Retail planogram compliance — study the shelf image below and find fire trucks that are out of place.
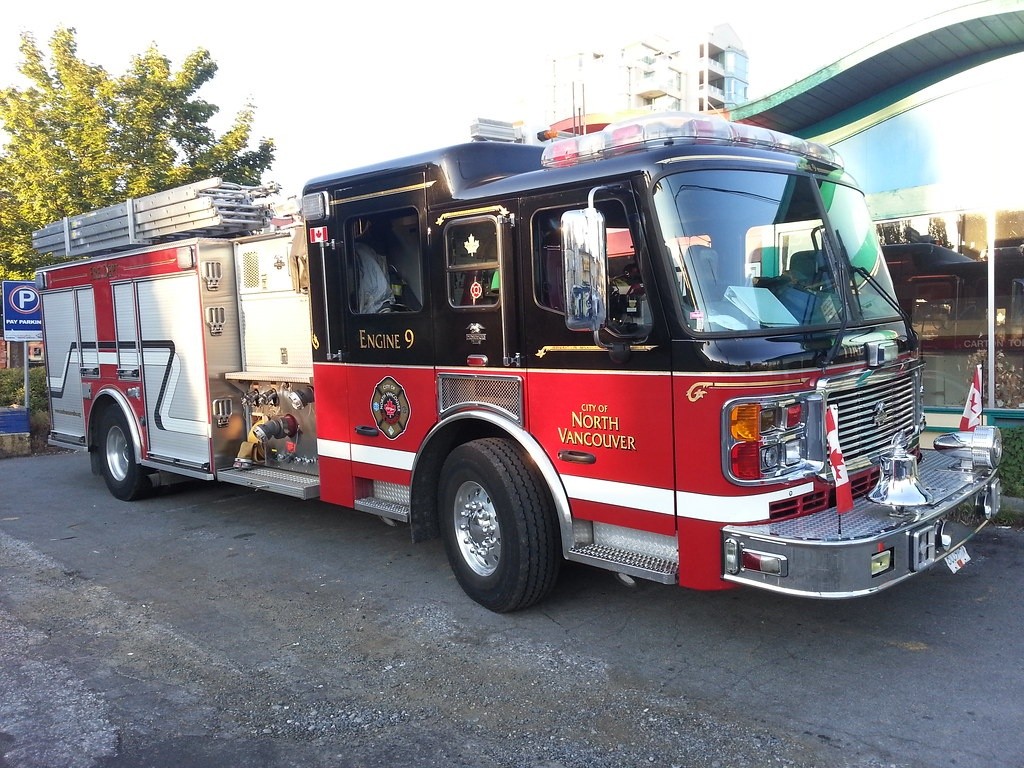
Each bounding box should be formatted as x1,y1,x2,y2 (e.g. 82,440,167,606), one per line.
29,110,1005,620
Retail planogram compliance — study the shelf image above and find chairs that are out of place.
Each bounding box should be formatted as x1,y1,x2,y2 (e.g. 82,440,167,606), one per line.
685,244,725,303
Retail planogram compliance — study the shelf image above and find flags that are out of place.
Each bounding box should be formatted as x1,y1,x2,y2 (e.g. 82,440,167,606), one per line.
824,404,854,516
960,363,983,432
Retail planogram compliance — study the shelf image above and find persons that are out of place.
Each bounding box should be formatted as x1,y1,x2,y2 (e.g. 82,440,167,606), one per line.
609,263,643,313
